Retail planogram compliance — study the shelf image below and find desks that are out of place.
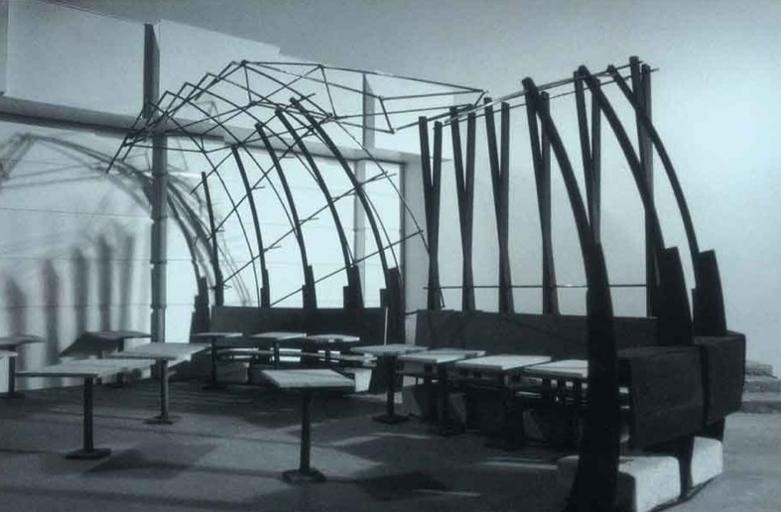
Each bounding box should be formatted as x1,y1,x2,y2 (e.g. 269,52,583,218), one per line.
195,333,363,398
2,331,46,400
398,331,588,460
259,369,355,484
89,331,153,390
16,356,157,459
108,341,212,425
349,343,429,425
